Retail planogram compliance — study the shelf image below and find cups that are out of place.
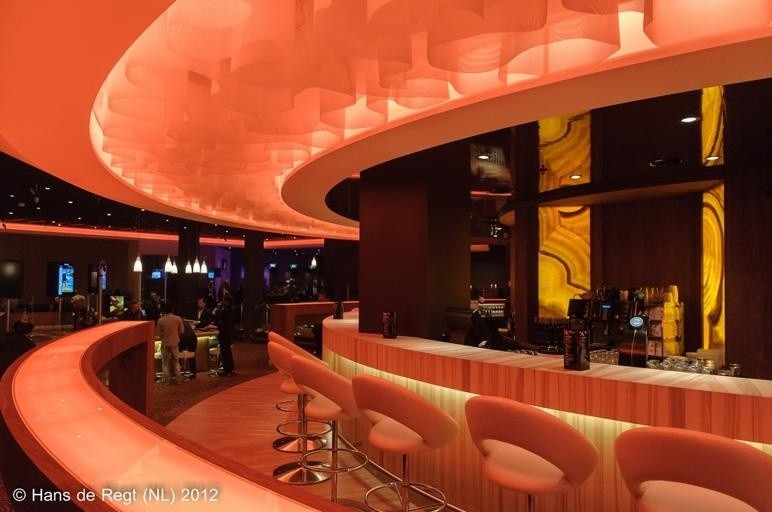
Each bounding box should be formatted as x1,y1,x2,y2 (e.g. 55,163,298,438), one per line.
589,348,619,365
646,356,716,375
383,311,397,338
619,283,679,304
562,328,590,370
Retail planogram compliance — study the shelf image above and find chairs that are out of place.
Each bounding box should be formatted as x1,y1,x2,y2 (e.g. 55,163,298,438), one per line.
349,371,462,511
464,393,602,511
292,356,368,511
267,328,328,455
616,419,772,511
265,341,332,485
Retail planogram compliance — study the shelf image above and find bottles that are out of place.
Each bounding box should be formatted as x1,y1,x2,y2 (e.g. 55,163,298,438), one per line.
728,363,742,376
333,298,343,320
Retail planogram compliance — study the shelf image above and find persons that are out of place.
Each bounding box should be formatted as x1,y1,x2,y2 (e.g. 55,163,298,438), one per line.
119,292,235,385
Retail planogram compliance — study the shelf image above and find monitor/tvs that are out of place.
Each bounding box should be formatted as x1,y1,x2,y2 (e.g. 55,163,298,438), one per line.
207,271,215,280
445,307,487,337
151,269,164,280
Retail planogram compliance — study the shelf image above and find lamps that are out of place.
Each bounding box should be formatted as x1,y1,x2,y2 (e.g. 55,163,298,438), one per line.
133,236,208,274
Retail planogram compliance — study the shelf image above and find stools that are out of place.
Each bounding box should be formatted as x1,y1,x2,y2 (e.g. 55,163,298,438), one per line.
208,347,225,377
173,351,196,378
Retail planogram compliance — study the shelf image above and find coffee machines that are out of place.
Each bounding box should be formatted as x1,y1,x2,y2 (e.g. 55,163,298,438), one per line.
567,298,686,367
